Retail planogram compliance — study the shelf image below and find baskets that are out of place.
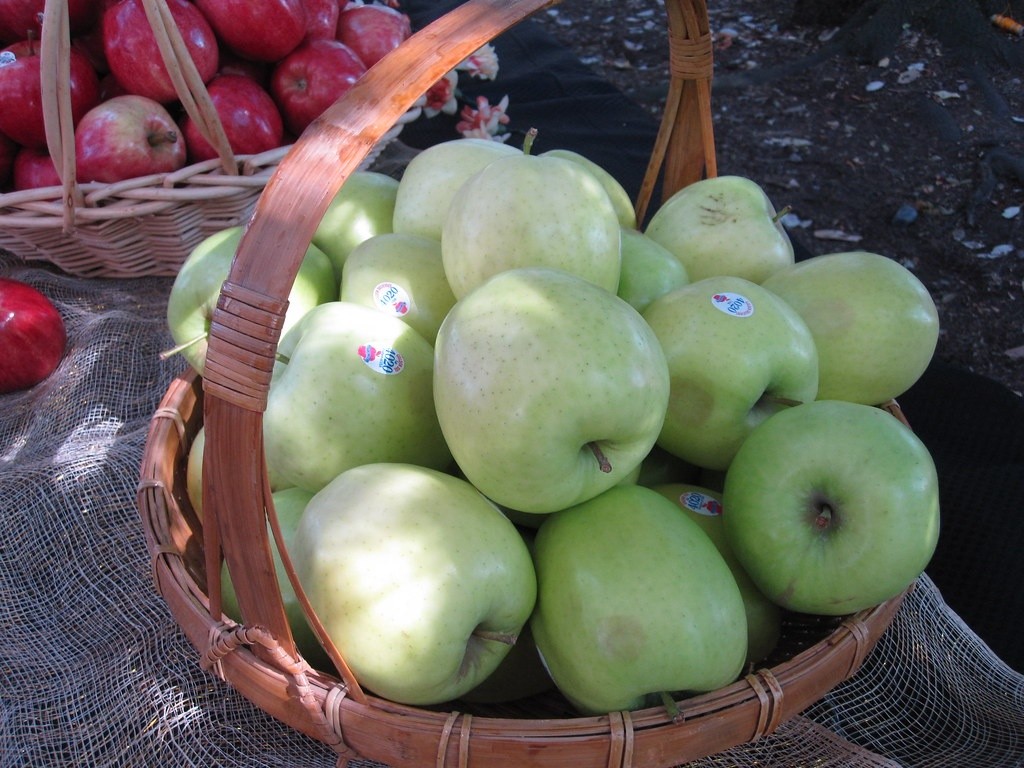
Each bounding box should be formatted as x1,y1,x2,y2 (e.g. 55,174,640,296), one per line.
0,0,427,280
137,0,911,768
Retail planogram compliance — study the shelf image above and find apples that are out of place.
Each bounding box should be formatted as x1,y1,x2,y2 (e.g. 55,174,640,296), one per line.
0,0,413,196
0,276,65,394
167,127,942,724
